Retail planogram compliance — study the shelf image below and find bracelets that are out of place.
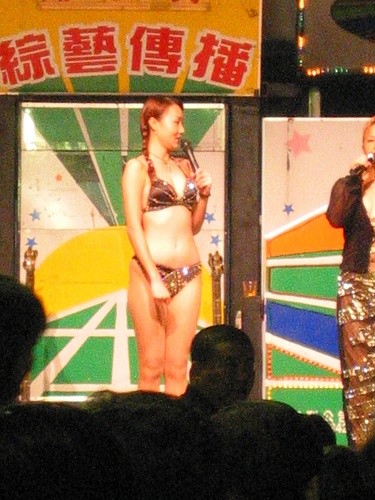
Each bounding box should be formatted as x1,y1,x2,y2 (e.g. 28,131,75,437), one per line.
199,193,211,198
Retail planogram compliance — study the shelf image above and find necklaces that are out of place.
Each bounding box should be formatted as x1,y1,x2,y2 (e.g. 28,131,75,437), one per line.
149,151,172,168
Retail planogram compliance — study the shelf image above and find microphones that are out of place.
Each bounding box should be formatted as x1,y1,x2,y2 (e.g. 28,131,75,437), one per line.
180,138,199,173
356,153,375,176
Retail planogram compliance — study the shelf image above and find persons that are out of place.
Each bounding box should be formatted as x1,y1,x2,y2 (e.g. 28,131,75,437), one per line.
325,116,375,449
121,96,211,397
0,273,375,500
